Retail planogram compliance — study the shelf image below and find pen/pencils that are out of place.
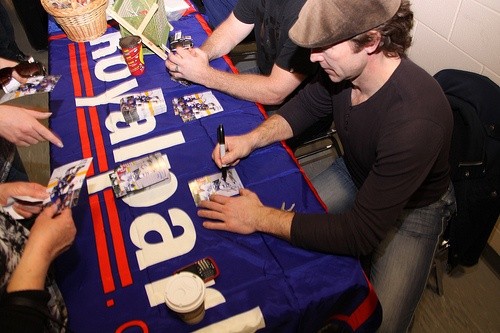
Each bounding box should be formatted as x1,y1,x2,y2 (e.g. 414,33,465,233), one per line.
217,123,227,183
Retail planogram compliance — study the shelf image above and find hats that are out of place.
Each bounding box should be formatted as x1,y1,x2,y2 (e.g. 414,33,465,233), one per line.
288,0,402,48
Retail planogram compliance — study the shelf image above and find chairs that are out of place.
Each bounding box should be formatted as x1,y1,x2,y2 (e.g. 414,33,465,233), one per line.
295,68,500,298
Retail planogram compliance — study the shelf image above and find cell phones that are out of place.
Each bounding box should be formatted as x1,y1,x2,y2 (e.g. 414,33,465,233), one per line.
173,257,219,283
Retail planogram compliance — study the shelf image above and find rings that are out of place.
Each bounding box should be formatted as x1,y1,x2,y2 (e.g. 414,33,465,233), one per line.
175,65,179,73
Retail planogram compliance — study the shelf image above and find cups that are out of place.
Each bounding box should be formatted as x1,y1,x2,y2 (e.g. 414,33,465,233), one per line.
119,36,146,77
164,271,206,325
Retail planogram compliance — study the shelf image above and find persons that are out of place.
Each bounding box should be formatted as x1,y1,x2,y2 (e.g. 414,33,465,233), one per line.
0,57,63,231
0,181,77,333
165,0,336,156
195,0,457,333
0,0,48,63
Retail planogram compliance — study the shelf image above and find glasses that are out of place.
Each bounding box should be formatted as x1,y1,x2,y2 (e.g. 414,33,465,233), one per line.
0,59,47,90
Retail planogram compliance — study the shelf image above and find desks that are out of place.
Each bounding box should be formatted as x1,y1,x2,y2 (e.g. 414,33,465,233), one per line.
44,0,385,333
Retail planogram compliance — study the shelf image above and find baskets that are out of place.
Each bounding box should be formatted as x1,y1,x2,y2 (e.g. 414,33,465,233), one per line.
40,0,110,43
